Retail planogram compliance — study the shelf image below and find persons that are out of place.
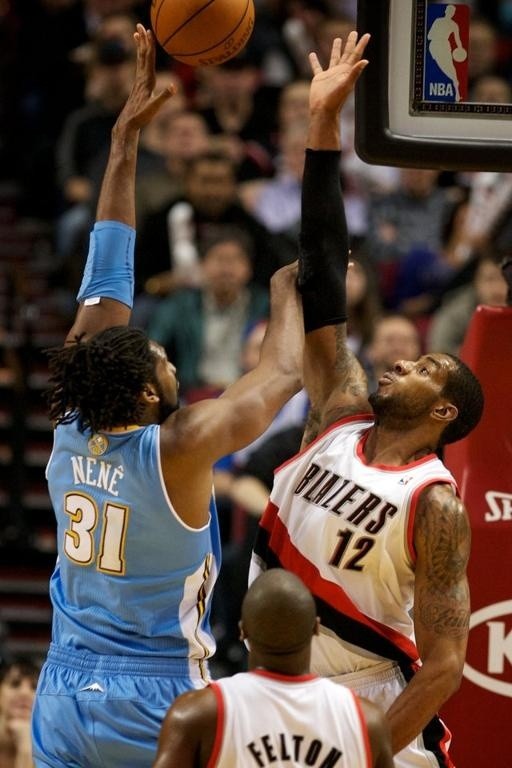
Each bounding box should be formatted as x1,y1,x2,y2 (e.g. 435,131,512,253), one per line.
1,2,512,768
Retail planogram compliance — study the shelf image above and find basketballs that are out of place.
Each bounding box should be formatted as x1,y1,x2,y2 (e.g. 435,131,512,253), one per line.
149,0,256,67
